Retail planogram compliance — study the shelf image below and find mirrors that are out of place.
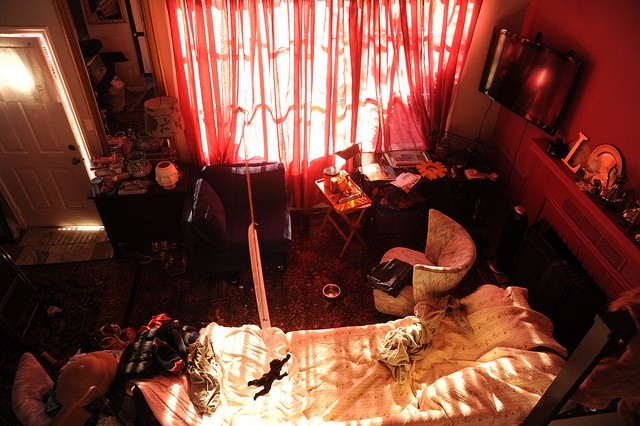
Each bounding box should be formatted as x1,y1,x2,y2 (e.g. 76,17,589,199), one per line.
53,0,189,164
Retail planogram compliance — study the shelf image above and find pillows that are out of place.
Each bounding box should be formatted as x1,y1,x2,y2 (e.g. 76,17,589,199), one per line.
188,179,227,242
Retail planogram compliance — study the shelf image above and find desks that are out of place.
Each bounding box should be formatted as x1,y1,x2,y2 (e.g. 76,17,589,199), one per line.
355,151,500,238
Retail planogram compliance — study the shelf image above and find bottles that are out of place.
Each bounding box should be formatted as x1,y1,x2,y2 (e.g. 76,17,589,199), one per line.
109,167,121,189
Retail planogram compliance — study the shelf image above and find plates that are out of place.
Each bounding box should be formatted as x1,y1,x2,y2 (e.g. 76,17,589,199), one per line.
587,143,625,184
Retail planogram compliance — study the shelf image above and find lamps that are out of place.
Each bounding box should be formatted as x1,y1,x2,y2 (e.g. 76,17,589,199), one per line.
142,97,185,159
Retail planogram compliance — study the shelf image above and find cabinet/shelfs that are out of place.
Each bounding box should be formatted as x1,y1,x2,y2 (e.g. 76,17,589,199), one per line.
89,163,192,260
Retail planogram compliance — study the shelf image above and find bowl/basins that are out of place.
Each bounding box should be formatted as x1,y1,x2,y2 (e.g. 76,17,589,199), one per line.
323,284,342,298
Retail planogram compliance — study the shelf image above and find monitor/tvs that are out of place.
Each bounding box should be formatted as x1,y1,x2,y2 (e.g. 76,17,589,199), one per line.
480,28,585,137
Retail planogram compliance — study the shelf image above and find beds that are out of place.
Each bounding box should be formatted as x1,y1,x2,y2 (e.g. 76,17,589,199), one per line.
127,284,564,426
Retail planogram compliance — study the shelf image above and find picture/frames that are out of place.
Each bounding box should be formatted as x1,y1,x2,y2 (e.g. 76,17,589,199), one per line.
82,0,127,25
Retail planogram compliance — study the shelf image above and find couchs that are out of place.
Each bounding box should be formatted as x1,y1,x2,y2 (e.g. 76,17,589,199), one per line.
373,208,476,316
182,162,292,274
